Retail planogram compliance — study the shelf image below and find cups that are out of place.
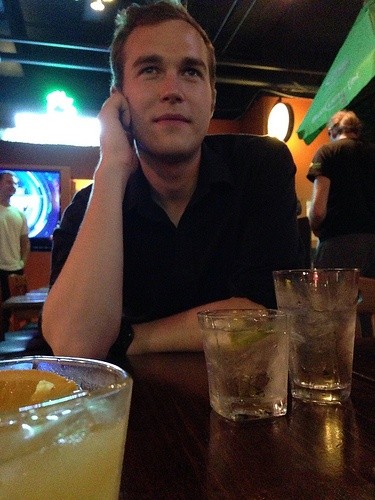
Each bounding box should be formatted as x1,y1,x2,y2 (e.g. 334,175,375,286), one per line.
272,267,362,406
196,307,291,422
0,355,134,500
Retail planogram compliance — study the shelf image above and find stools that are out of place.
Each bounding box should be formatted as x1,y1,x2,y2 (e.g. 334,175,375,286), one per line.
0,331,34,354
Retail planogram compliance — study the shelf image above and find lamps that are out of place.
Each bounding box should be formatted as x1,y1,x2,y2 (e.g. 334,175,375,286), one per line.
266,100,293,144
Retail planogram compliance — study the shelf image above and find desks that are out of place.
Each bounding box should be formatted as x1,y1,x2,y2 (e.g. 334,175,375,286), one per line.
3,286,49,310
106,354,375,500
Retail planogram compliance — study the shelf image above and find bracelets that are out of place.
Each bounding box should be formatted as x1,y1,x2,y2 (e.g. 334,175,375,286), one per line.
106,315,135,362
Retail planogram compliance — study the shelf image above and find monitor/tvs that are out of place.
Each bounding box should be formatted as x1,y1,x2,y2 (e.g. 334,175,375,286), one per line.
0,164,71,252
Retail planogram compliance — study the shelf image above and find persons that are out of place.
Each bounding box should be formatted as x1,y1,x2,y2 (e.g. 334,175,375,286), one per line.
0,170,30,340
38,0,308,359
305,110,375,280
